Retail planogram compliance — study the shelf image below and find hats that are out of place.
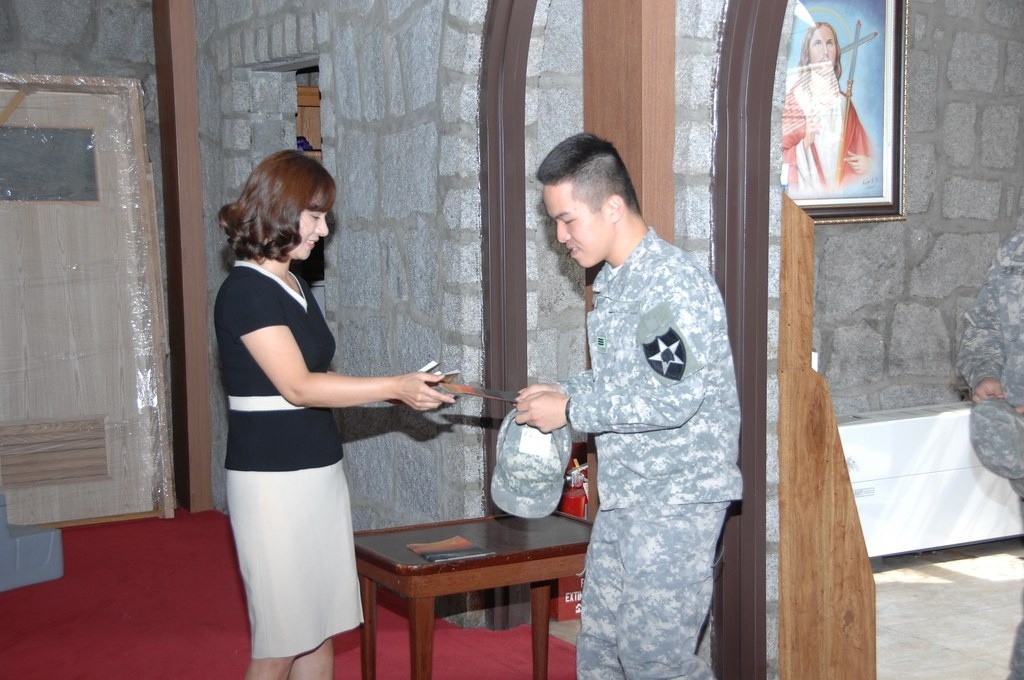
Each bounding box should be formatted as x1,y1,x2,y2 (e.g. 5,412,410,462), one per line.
490,408,572,519
969,399,1024,498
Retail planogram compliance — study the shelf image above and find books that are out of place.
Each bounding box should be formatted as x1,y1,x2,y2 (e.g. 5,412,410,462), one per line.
438,382,519,405
406,534,496,563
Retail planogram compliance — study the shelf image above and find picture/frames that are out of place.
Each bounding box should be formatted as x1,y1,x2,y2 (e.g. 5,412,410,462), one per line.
780,1,910,227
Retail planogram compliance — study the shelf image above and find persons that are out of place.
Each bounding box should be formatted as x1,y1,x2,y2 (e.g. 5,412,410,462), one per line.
213,151,457,679
955,207,1024,678
513,133,747,680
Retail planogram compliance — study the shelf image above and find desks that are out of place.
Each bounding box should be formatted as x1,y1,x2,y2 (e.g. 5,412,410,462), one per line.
353,510,594,680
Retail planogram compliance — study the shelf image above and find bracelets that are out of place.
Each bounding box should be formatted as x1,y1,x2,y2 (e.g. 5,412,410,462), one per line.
565,397,572,425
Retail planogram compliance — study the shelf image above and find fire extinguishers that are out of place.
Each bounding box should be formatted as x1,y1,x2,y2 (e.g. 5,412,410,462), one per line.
558,463,589,521
